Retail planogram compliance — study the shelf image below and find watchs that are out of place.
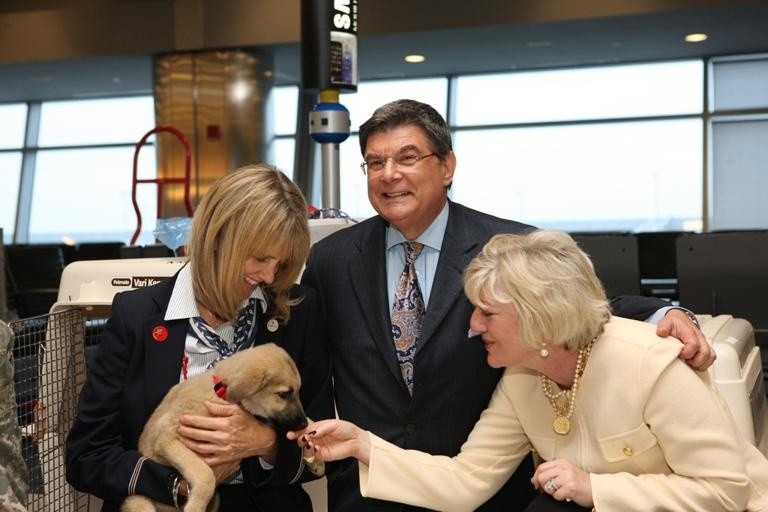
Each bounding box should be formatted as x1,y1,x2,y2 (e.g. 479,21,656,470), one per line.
685,311,698,327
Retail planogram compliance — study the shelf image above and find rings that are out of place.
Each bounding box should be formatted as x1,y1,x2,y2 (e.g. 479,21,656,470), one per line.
549,479,558,492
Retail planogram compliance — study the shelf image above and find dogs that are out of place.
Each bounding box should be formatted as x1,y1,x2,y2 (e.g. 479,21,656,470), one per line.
119,341,308,512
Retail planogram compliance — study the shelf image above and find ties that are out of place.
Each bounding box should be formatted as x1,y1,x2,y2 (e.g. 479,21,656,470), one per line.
391,242,425,396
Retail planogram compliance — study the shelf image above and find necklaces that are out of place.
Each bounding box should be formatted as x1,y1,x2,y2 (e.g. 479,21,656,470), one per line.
541,332,597,435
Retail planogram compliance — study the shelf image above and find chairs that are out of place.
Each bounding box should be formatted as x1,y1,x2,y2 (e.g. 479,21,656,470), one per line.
2,242,175,319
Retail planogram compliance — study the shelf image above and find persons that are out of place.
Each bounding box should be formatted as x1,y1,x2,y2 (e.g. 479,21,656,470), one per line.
302,100,716,512
287,231,768,511
64,165,334,512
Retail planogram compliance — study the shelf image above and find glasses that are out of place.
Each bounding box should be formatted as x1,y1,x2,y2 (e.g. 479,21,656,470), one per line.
360,153,436,176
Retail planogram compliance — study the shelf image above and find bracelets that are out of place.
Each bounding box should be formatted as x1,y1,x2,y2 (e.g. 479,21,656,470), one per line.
172,473,182,509
